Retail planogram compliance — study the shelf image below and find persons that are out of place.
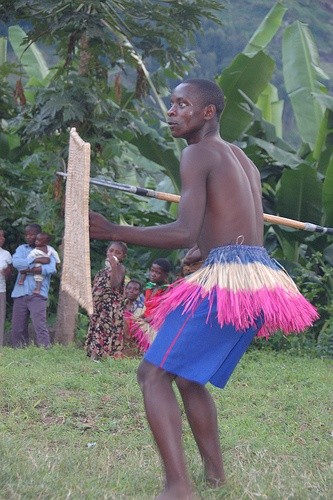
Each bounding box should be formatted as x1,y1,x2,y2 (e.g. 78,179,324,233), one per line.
57,80,333,500
0,224,12,347
18,232,51,295
12,223,61,349
85,241,128,360
125,259,170,356
